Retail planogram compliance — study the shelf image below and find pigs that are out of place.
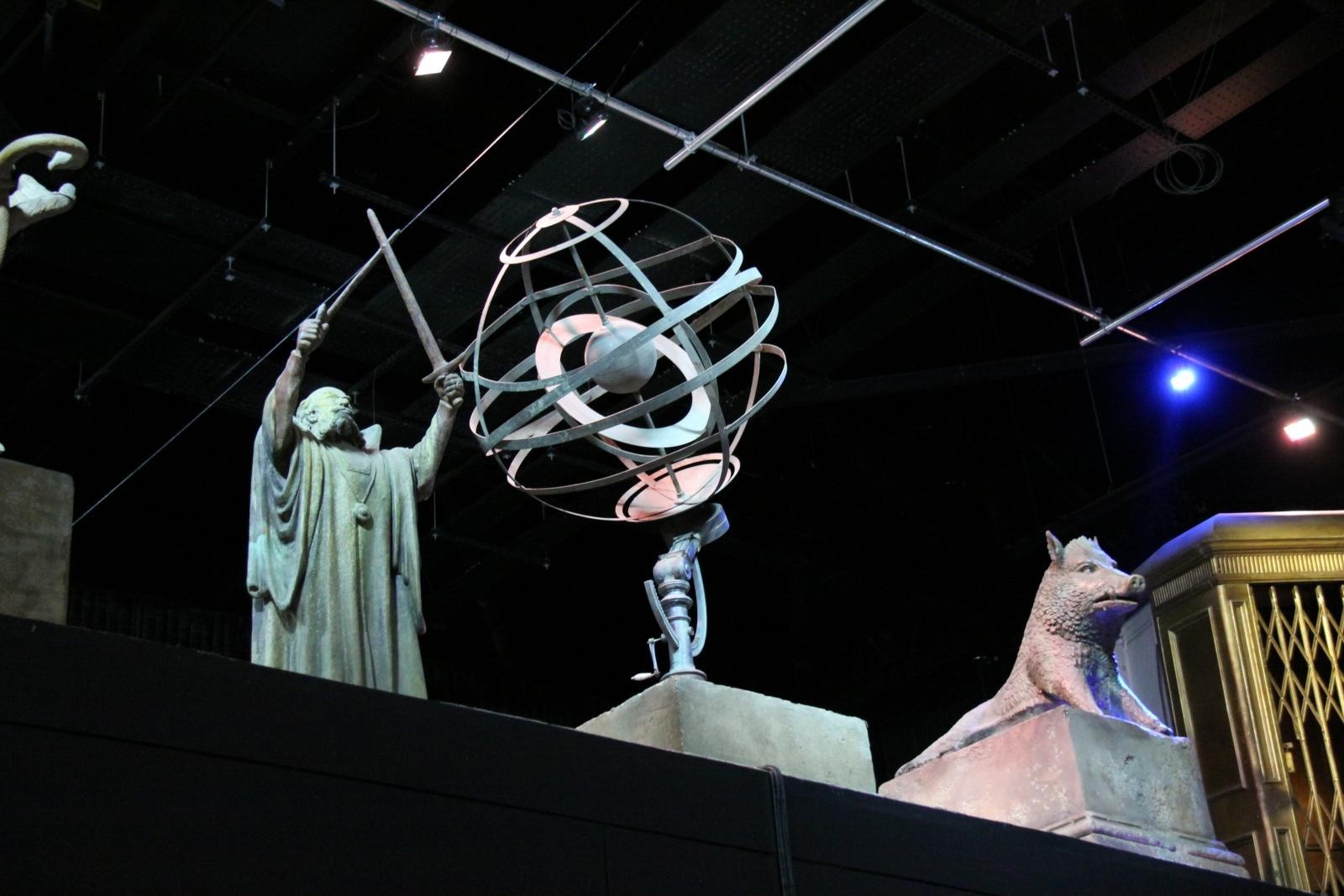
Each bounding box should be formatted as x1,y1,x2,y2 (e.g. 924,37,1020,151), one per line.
894,530,1175,777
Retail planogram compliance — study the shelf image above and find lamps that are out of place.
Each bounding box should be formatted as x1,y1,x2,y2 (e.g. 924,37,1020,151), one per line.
411,13,453,76
557,77,610,141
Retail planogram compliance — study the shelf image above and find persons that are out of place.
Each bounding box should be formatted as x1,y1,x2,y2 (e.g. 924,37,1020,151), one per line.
244,316,466,703
